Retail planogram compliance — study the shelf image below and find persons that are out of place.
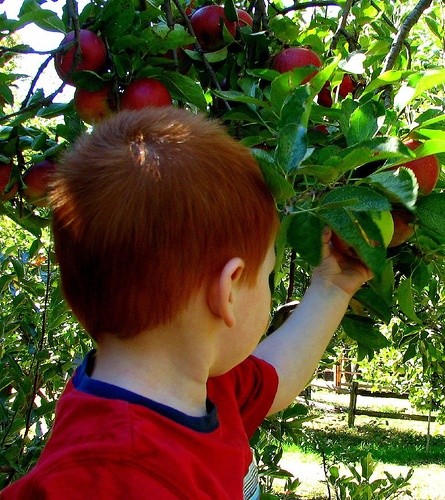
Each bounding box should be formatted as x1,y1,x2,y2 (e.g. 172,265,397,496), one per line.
0,107,375,500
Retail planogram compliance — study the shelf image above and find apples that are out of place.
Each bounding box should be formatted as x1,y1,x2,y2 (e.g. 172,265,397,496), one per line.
74,83,117,125
186,6,253,52
273,47,355,134
0,160,20,200
119,77,172,114
22,160,63,207
328,140,439,258
55,29,104,87
